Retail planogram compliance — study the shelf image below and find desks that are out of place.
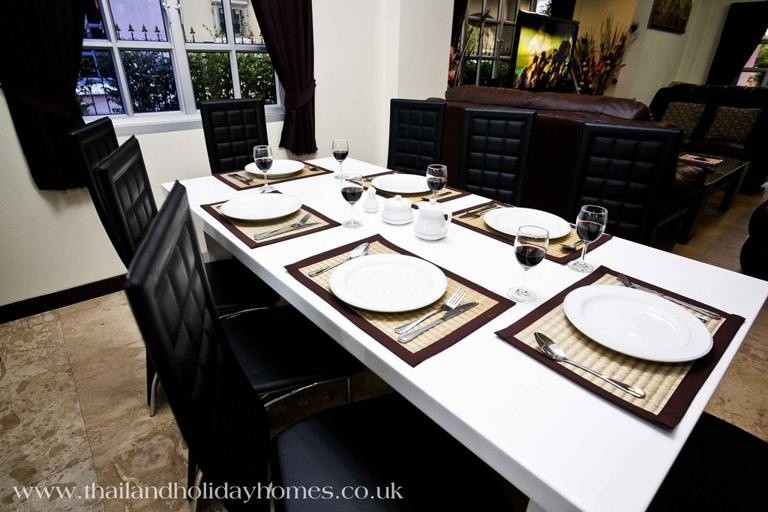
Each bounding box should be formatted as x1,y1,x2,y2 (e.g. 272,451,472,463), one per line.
641,129,751,208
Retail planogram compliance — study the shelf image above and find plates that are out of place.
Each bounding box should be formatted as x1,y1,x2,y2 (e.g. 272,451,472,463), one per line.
220,158,714,362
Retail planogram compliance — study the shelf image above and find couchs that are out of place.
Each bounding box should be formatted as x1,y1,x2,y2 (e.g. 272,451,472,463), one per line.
447,92,707,238
738,200,768,279
646,80,764,193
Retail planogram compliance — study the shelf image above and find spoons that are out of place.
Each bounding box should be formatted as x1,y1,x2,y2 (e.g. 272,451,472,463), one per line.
532,331,646,398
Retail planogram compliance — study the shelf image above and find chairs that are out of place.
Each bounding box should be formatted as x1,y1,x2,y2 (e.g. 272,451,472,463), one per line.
645,399,768,512
111,178,390,512
197,97,266,174
388,100,444,176
92,133,163,267
463,104,535,209
69,113,117,186
583,122,679,257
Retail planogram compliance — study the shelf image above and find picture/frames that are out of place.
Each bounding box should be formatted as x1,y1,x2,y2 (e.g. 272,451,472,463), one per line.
647,1,691,34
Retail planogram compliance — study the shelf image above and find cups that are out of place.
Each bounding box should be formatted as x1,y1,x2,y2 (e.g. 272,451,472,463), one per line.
364,184,452,241
